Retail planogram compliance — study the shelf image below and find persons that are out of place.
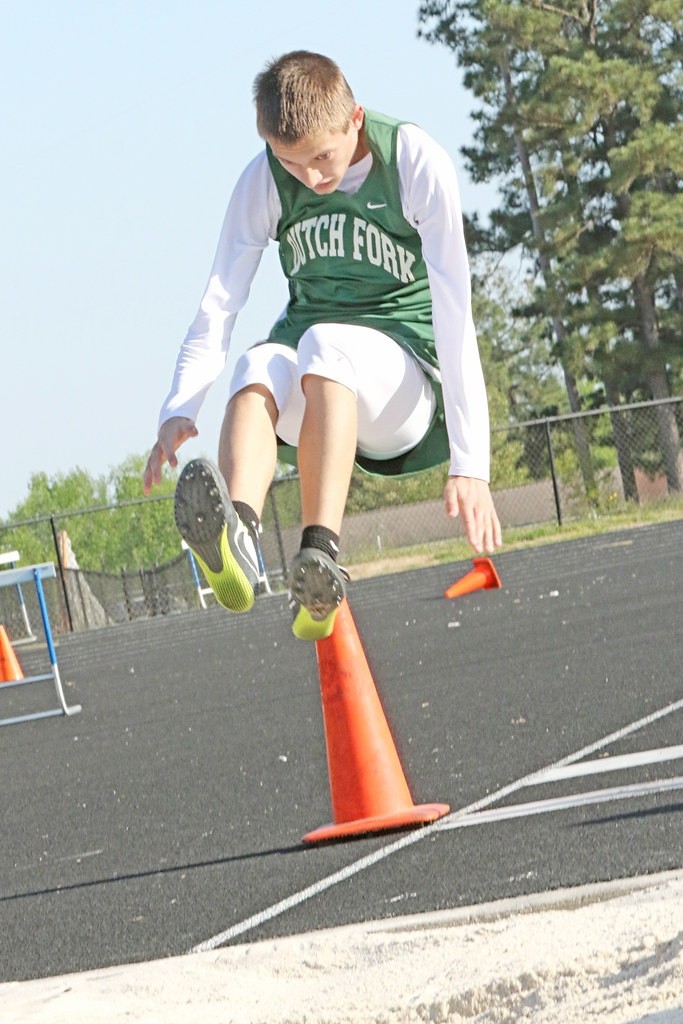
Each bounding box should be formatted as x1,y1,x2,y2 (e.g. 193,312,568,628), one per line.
144,51,502,639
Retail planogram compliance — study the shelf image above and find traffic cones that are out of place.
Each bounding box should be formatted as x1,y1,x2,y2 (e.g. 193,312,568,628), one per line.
444,557,504,599
302,597,450,844
0,625,26,684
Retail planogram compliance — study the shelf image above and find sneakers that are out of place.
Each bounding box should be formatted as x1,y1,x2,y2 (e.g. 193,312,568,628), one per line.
284,550,345,637
178,458,273,614
